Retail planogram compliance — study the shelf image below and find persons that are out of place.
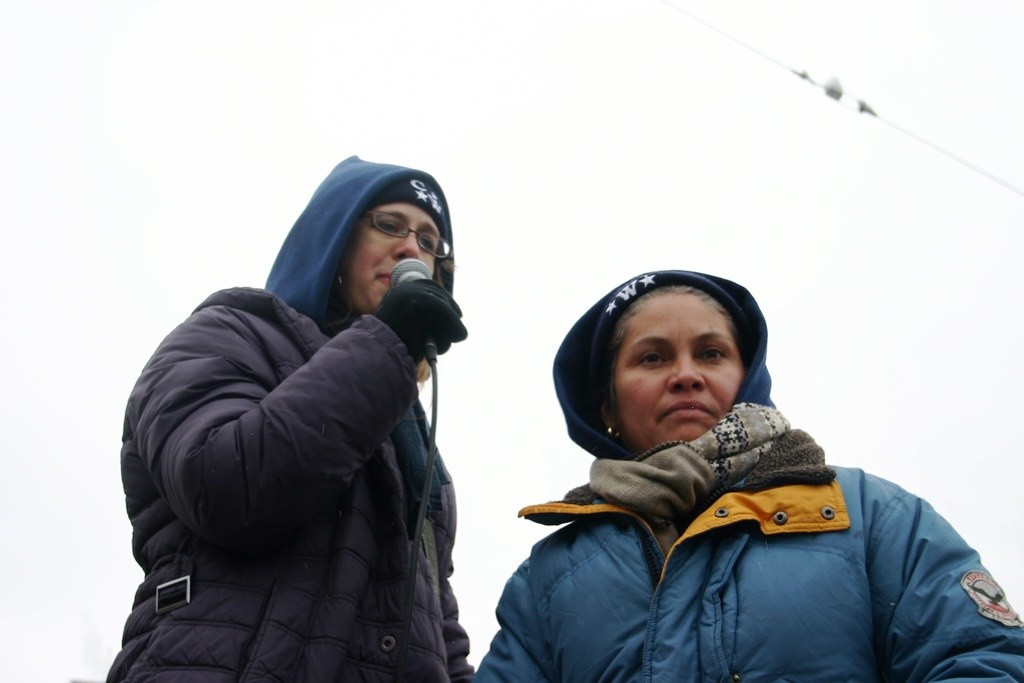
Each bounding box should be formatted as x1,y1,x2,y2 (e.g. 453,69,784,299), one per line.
105,156,476,683
471,270,1024,683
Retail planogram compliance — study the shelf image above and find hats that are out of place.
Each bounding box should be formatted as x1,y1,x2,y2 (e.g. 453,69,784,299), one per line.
582,271,755,352
366,173,453,246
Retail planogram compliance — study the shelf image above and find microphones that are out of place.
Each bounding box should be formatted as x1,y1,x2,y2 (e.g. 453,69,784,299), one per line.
389,257,437,365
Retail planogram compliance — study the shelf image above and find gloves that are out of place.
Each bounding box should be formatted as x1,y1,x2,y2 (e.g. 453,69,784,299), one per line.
372,278,467,353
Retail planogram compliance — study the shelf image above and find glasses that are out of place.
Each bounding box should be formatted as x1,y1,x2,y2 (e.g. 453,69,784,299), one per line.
365,210,452,258
